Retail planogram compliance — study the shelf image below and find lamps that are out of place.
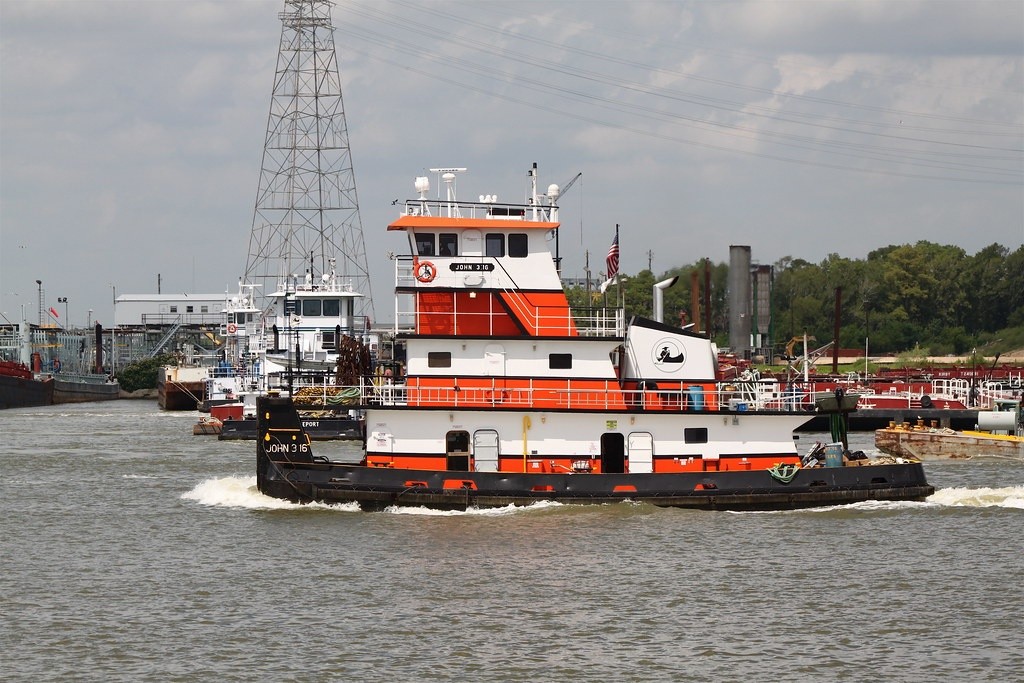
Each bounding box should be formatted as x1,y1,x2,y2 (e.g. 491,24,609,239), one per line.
529,198,533,204
528,169,532,176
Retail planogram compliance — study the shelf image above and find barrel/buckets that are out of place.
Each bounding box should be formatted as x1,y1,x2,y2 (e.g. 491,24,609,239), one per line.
721,386,736,405
825,445,844,468
687,386,704,410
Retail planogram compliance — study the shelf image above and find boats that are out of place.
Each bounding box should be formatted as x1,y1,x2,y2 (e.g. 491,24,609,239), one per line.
245,160,938,515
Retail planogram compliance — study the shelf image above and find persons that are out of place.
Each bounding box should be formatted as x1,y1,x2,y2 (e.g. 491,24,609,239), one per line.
787,361,792,371
782,368,785,373
245,413,257,420
229,416,233,420
746,368,753,376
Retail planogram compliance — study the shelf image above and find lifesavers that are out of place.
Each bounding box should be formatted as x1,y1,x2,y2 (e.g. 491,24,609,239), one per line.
414,260,437,283
228,324,236,333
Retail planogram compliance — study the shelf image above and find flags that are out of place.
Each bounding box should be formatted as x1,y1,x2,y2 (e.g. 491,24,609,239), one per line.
605,232,620,279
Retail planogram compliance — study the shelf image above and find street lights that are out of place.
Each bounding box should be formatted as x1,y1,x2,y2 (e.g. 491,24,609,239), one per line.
36,280,42,325
87,309,93,328
971,347,978,406
